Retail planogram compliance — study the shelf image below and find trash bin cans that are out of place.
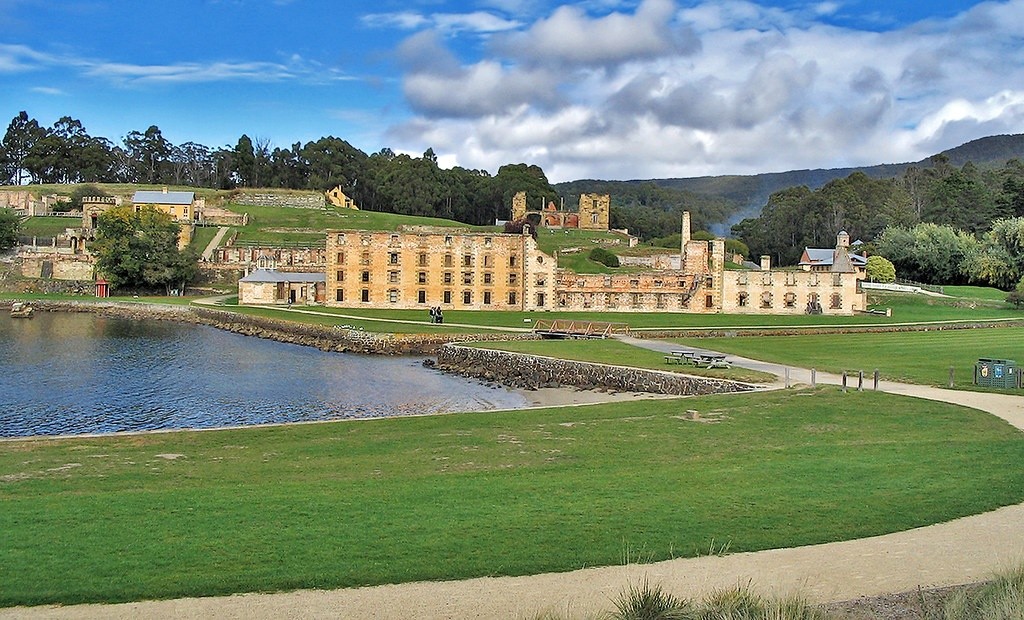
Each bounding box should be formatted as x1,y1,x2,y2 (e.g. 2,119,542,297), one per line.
95,279,111,298
974,358,1017,389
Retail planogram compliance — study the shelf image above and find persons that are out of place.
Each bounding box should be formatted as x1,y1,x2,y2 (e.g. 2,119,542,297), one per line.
287,296,294,311
429,306,444,323
